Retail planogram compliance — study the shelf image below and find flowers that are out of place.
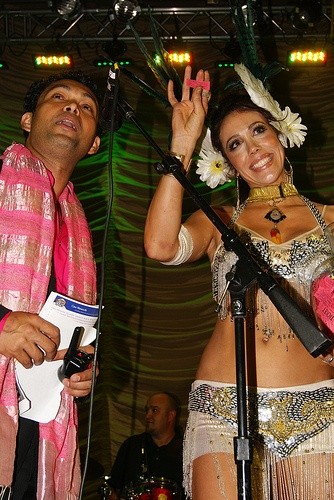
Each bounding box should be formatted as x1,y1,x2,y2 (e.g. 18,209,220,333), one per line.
189,123,231,187
233,62,309,149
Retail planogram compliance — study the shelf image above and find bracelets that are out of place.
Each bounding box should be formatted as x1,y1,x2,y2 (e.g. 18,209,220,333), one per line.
165,150,193,181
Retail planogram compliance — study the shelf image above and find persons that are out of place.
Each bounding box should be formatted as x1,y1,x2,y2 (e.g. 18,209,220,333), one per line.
144,65,333,499
104,391,189,499
0,72,102,500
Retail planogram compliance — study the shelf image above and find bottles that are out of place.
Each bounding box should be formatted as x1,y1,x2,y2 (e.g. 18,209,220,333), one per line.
97,477,112,500
126,475,178,500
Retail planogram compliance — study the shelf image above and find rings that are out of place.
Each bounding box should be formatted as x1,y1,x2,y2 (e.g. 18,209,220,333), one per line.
323,359,328,363
203,91,211,101
327,357,334,363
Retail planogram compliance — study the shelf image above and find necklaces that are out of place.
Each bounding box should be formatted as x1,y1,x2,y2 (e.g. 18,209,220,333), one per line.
247,182,299,244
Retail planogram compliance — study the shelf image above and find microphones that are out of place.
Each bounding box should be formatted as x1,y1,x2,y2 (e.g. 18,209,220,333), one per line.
100,63,122,131
